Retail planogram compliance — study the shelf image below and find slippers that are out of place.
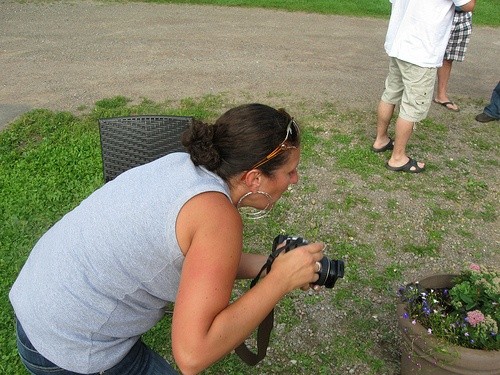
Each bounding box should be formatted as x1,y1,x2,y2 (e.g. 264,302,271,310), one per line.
370,136,395,152
386,157,425,173
435,98,459,111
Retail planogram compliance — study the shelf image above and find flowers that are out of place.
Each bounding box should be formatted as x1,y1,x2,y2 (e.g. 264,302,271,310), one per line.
397,265,500,367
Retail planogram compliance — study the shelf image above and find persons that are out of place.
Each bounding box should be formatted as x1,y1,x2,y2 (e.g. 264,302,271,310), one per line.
433,7,472,112
474,80,500,123
9,102,325,375
370,0,477,172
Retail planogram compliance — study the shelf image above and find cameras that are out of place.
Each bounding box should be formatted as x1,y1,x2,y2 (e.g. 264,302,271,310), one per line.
284,237,345,288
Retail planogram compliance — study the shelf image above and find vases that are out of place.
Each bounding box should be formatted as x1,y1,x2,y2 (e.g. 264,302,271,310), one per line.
396,274,500,375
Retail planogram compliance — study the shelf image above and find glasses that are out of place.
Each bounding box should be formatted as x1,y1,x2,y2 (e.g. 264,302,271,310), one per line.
240,108,301,180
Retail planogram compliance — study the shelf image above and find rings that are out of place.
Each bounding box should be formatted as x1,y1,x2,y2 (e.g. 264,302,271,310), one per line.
316,262,322,273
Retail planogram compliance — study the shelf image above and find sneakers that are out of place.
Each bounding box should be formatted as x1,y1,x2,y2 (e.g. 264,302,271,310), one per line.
475,113,498,122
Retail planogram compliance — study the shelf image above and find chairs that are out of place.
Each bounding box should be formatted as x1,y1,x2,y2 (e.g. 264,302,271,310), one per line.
97,115,190,314
